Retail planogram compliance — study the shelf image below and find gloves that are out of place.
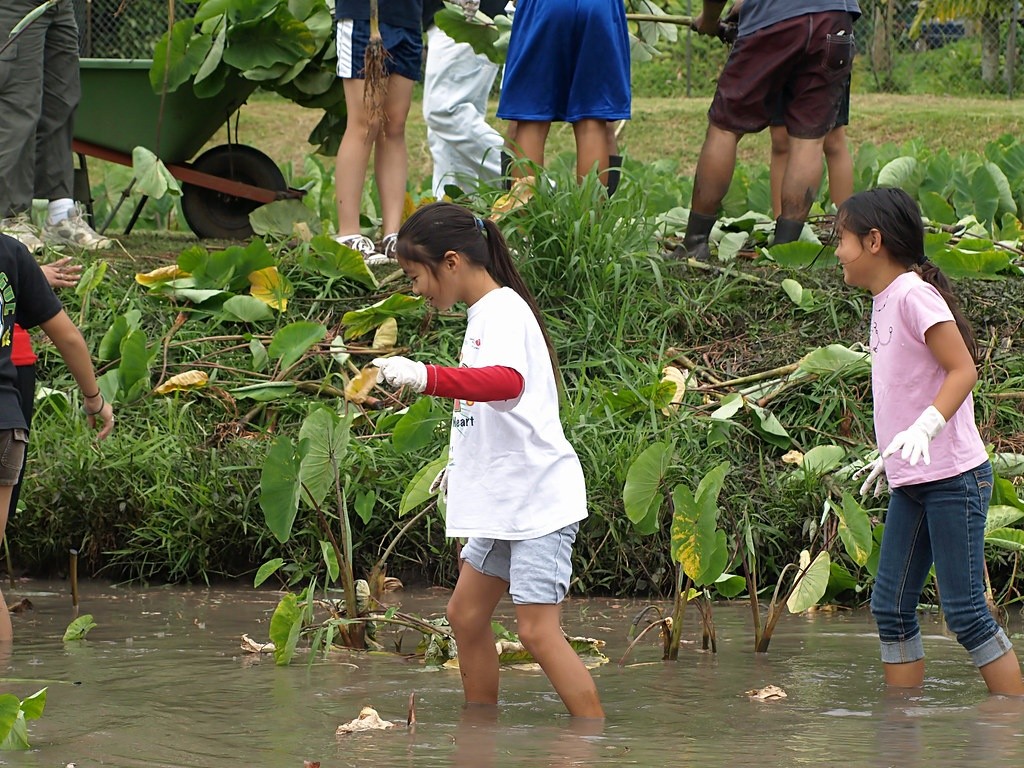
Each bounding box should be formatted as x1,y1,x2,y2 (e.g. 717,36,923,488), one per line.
371,356,428,394
882,405,947,466
450,0,480,23
852,456,887,497
429,467,448,505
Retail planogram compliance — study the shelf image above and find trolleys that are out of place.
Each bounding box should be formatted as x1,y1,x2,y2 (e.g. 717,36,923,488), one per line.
69,55,312,245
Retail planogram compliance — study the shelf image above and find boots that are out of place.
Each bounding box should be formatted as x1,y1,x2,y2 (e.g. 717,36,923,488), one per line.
664,211,719,262
768,215,804,249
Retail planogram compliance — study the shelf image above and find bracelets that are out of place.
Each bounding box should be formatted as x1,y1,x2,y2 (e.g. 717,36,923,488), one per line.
82,387,104,415
430,364,438,396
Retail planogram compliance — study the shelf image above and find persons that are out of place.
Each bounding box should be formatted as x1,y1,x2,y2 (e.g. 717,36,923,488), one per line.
422,0,632,257
333,0,423,265
0,0,114,254
0,231,114,768
658,0,862,264
833,187,1024,697
371,202,606,719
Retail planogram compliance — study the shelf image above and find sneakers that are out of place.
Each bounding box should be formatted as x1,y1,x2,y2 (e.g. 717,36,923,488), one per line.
381,235,399,264
39,200,108,250
0,217,44,253
342,236,389,265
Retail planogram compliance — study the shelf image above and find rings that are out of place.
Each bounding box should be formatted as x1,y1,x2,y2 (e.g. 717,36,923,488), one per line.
112,416,114,422
60,273,64,279
